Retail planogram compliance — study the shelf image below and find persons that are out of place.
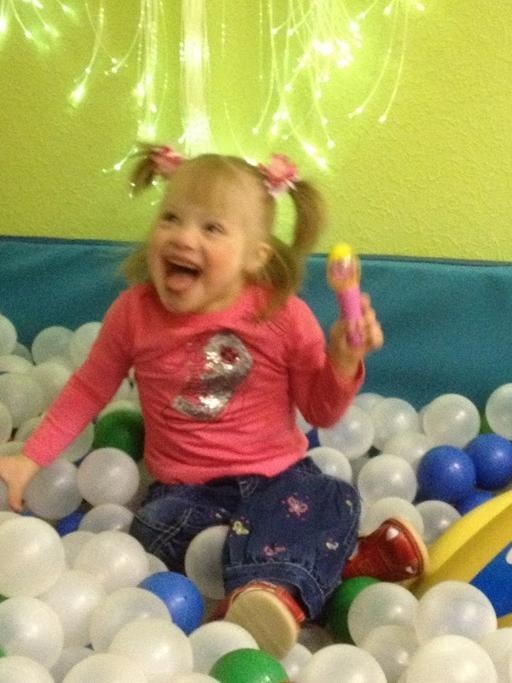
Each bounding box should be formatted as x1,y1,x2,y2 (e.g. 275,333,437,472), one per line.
0,140,425,659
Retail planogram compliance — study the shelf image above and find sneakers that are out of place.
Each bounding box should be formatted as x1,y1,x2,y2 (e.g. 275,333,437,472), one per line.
206,579,306,661
341,516,429,583
